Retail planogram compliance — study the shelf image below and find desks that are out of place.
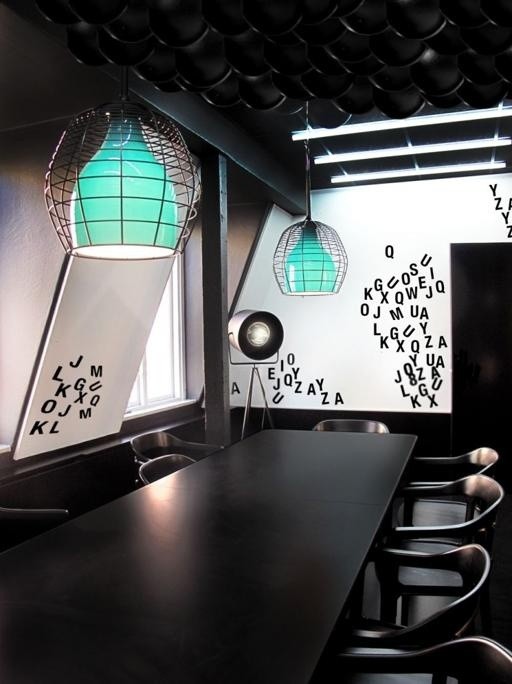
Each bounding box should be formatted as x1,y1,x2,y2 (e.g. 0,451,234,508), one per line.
2,430,418,682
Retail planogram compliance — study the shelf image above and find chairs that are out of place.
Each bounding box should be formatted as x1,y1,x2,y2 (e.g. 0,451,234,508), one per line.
2,508,68,549
130,431,224,470
333,447,511,683
133,453,198,489
314,422,390,434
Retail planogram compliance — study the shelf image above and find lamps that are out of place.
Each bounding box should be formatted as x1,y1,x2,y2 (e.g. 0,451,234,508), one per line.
44,1,201,259
227,308,284,441
272,98,348,296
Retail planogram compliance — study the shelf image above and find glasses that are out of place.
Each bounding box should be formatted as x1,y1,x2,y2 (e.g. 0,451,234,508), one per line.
227,308,284,441
